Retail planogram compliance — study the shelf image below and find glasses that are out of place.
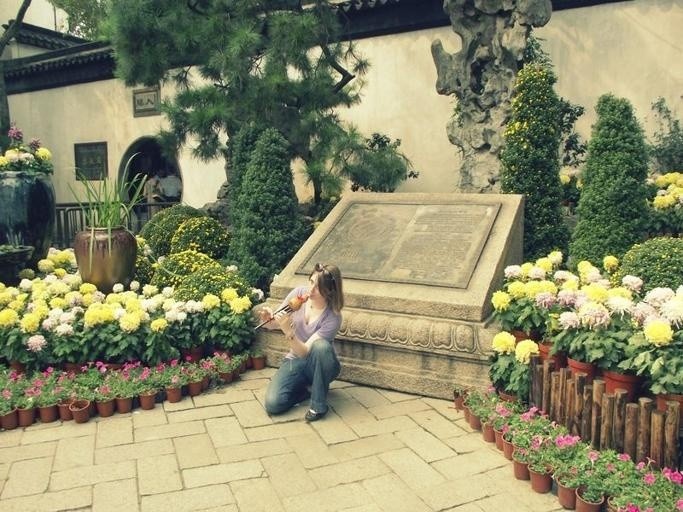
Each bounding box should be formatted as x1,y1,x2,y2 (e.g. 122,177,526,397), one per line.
316,263,334,280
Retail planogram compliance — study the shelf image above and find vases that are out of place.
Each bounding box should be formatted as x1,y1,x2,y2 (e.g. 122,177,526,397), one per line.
509,329,682,416
0,399,90,430
453,390,622,510
0,164,56,269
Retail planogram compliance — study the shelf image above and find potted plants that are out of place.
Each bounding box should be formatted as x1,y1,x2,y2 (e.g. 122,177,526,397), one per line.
0,230,35,287
62,162,181,293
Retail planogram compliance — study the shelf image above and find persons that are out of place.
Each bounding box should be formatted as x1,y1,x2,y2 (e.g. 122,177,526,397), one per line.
149,167,182,203
257,262,343,421
142,166,161,219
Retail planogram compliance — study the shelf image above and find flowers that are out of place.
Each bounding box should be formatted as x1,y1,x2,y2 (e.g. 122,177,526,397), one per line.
491,328,542,378
483,251,683,347
0,248,263,414
1,120,52,168
488,386,682,511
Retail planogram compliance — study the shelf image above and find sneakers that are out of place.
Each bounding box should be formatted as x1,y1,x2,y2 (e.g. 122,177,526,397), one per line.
305,403,329,420
